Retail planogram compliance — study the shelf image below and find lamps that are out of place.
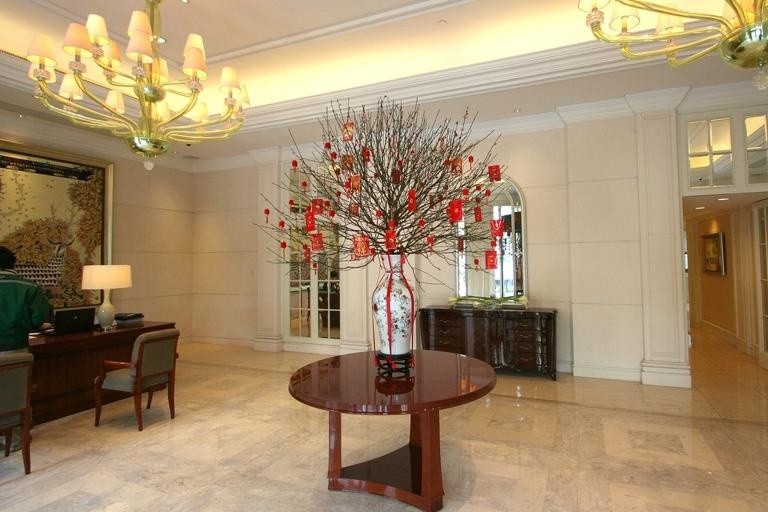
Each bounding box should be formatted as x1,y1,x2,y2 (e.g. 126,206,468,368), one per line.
25,0,251,171
577,0,767,91
81,264,131,330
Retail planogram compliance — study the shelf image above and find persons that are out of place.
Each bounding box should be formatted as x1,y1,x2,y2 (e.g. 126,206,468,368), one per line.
29,302,55,333
0,247,48,351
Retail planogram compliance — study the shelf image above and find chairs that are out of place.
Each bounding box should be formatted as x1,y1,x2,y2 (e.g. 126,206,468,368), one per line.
0,353,34,475
319,290,340,326
94,329,180,431
307,287,321,320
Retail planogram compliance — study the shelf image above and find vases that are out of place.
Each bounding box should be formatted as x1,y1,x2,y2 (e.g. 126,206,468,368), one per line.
373,255,418,356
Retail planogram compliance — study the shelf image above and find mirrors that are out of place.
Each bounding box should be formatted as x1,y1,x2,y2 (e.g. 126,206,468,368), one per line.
455,173,527,309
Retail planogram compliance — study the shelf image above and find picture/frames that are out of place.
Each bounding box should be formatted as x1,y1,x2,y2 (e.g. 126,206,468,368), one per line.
290,203,339,290
701,232,726,276
0,138,114,316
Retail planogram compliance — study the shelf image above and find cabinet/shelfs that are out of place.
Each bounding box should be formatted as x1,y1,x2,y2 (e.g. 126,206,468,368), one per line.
419,309,557,381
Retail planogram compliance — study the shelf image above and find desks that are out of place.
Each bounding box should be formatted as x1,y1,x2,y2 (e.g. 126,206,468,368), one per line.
28,320,176,429
289,350,496,512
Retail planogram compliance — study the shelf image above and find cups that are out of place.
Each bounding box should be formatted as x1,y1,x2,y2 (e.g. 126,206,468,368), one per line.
456,354,469,378
457,376,469,396
